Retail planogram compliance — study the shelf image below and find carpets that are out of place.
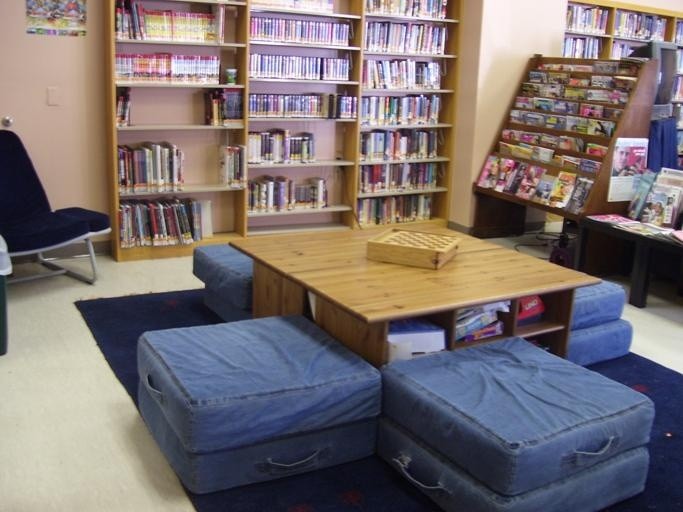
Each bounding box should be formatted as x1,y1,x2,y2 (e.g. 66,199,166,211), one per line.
74,285,683,512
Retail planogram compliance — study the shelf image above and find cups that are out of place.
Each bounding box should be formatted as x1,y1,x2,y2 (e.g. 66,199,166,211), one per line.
225,68,238,85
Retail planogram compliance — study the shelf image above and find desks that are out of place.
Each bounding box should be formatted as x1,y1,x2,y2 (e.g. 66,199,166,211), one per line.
228,224,602,369
577,212,683,309
0,232,13,355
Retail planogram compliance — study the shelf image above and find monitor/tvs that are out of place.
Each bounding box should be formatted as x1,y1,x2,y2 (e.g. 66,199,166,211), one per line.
629,40,677,121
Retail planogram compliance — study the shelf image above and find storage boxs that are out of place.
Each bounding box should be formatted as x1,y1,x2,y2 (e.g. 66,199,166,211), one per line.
386,317,446,365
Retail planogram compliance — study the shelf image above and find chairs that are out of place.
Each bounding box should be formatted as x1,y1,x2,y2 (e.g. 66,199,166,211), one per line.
0,130,112,284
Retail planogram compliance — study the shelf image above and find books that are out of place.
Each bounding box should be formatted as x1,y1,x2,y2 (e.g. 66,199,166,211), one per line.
247,1,357,116
478,1,683,245
118,141,184,193
116,87,132,126
116,1,226,42
360,1,448,225
387,301,513,355
250,130,316,164
248,176,328,214
205,88,244,125
116,53,220,85
218,144,247,186
119,198,212,248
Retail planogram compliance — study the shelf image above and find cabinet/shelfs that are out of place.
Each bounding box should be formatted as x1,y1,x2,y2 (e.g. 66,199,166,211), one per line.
472,53,659,271
104,0,464,261
565,1,683,103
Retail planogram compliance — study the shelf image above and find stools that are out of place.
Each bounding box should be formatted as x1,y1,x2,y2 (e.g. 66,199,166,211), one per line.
135,314,382,498
378,336,656,512
565,278,632,369
192,245,256,324
